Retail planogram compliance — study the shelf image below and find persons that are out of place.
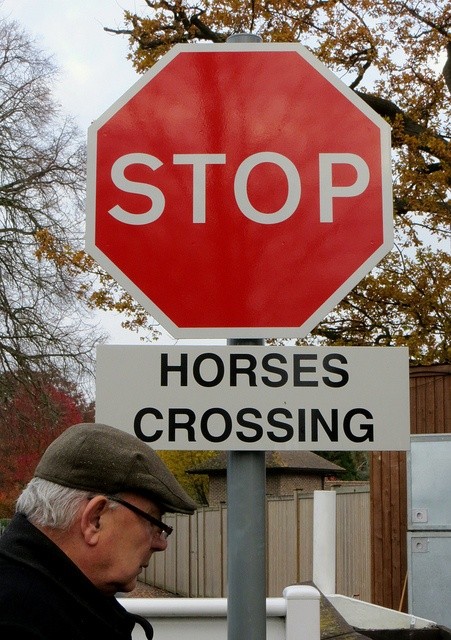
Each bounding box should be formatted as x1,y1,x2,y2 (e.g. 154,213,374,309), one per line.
0,422,196,640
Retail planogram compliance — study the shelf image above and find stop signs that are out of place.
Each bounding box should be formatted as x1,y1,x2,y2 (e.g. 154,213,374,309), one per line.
83,42,394,341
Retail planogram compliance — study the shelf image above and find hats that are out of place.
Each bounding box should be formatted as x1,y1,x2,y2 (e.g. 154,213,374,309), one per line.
33,422,198,514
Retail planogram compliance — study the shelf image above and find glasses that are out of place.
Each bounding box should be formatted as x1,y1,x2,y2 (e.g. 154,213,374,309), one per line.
86,494,173,541
